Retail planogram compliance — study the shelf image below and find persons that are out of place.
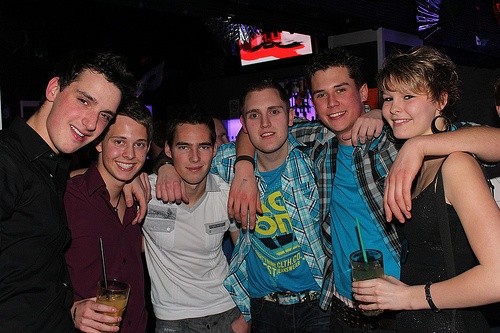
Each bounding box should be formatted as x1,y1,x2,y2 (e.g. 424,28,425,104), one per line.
71,106,251,333
352,46,500,333
0,46,134,333
65,101,149,333
156,80,385,333
228,51,500,333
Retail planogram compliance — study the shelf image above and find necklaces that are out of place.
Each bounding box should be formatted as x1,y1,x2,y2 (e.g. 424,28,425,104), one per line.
113,192,122,212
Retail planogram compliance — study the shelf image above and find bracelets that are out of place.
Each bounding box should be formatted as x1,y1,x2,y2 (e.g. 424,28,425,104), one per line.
233,156,255,171
425,282,439,311
73,307,77,320
157,162,173,174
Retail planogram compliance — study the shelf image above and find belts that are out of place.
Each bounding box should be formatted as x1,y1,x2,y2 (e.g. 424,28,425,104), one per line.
331,297,371,327
265,289,319,304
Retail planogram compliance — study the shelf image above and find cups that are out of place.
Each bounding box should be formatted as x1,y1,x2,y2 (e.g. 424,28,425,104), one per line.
350,249,384,316
96,280,130,333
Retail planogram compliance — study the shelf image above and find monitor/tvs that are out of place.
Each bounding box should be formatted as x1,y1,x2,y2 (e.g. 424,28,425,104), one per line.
226,24,318,86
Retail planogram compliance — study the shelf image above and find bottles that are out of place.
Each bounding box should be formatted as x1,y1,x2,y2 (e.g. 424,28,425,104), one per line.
284,76,308,120
